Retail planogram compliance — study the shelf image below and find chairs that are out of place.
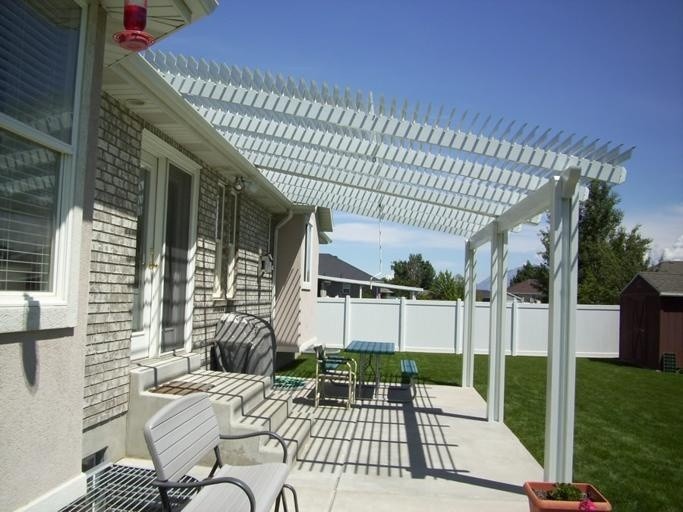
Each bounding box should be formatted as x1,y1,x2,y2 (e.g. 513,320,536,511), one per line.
314,342,357,409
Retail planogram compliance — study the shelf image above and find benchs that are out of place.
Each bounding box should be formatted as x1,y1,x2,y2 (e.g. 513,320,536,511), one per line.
324,355,351,386
143,391,298,512
389,360,418,402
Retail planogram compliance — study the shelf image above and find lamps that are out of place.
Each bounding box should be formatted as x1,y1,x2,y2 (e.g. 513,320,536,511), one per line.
233,177,244,190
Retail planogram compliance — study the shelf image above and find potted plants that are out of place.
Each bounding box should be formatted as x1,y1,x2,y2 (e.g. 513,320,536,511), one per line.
524,481,611,512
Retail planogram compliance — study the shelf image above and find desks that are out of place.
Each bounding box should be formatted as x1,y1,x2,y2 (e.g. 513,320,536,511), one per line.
344,340,394,400
